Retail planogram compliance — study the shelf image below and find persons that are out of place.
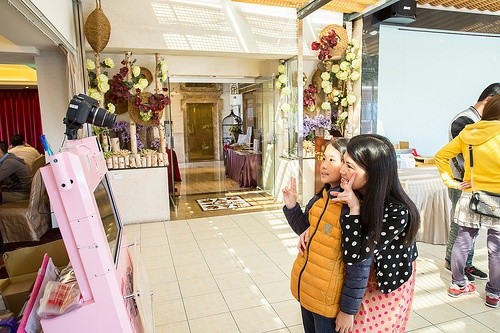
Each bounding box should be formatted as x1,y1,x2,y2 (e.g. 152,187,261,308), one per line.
282,139,374,332
0,141,32,206
444,83,499,283
433,92,499,306
330,135,422,332
7,133,40,167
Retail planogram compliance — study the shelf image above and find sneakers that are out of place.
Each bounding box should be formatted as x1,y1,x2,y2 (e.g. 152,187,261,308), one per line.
464,266,488,279
444,258,475,282
485,292,500,308
448,284,477,298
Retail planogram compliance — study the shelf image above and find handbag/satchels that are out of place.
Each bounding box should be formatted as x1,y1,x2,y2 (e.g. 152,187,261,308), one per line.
469,190,500,219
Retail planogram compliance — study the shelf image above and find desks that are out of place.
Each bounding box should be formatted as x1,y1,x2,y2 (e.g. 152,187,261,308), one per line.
225,149,262,188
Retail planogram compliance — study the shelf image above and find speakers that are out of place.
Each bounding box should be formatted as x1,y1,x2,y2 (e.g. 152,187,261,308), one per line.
371,0,416,28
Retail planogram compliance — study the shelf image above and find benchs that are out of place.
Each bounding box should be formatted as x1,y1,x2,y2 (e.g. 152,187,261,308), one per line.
0,239,70,314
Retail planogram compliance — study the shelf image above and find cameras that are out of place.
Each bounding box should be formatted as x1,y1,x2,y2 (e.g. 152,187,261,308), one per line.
66,93,117,129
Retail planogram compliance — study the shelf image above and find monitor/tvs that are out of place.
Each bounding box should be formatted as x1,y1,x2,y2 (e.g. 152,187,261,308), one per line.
93,172,122,269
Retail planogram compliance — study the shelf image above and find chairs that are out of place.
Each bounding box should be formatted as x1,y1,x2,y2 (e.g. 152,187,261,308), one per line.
0,155,52,243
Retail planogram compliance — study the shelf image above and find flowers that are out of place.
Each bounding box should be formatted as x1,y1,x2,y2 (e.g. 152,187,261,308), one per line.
303,29,361,137
86,57,172,151
